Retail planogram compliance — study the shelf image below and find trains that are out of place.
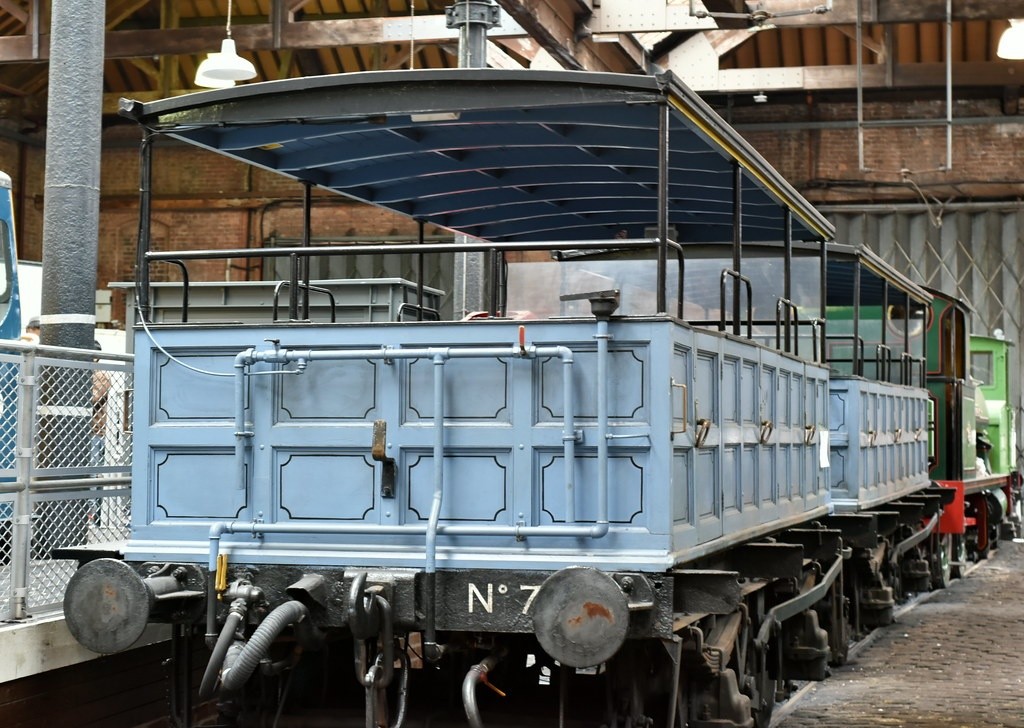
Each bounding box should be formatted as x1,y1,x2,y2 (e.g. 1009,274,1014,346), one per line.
47,65,1024,727
